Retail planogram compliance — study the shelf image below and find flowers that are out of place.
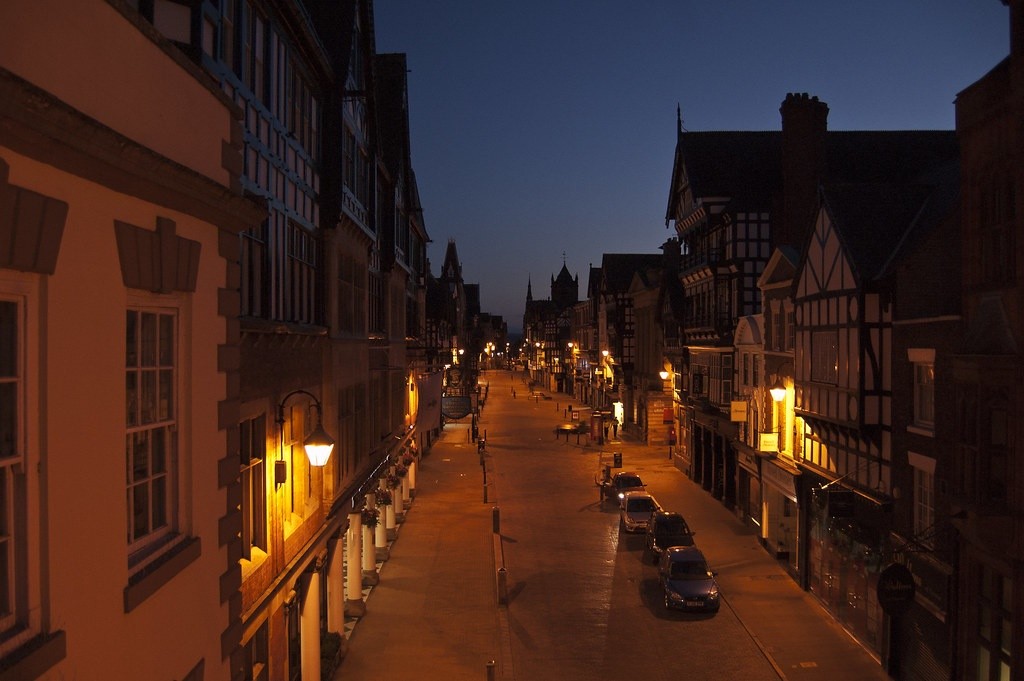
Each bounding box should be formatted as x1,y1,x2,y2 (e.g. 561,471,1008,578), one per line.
360,446,419,527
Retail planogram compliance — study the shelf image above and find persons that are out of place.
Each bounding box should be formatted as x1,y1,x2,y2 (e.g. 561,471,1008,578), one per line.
611,416,619,439
599,470,606,481
604,419,610,437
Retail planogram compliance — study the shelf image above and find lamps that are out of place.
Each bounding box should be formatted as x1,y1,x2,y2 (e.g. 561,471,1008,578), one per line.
658,351,682,380
273,389,336,490
444,336,615,374
768,360,795,402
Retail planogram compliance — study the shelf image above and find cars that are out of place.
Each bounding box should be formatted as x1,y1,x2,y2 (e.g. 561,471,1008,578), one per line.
602,468,647,502
618,488,662,532
657,544,724,611
644,510,699,559
